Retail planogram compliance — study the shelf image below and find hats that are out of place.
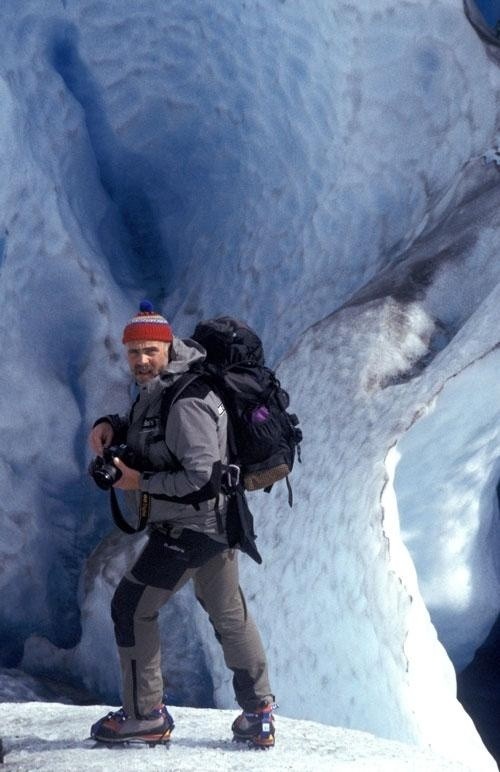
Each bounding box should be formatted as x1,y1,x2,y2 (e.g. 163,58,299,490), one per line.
121,300,173,344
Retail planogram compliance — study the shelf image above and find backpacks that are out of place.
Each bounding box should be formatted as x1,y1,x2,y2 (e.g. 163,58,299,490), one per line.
157,315,303,493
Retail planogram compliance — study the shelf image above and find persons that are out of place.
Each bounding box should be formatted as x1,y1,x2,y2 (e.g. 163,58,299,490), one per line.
87,312,277,750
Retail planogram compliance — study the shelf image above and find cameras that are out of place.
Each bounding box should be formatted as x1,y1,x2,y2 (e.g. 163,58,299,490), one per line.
89,445,134,492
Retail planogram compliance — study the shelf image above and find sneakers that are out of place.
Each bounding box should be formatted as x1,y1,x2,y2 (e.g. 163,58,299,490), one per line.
230,711,274,746
87,707,175,743
